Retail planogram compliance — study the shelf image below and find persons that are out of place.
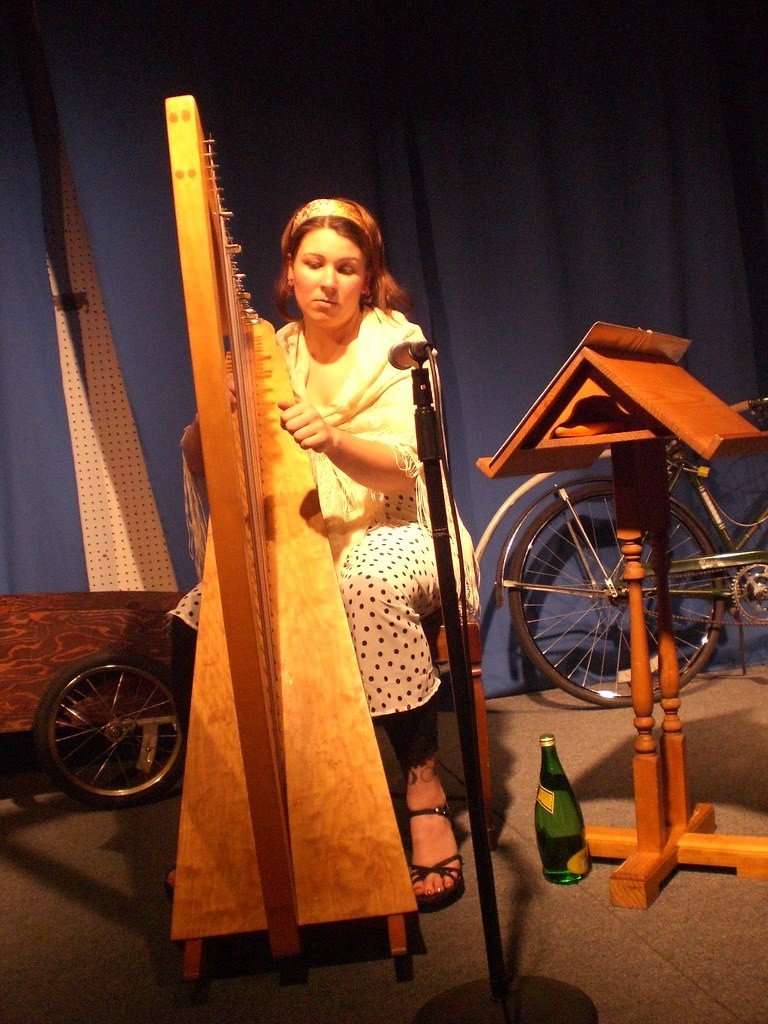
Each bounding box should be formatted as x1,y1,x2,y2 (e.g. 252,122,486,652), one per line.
165,196,483,906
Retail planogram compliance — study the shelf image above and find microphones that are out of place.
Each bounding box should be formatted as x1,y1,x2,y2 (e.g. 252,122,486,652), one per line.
387,341,434,370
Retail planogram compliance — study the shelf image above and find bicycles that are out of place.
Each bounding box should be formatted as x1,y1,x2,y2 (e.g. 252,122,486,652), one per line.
494,395,768,709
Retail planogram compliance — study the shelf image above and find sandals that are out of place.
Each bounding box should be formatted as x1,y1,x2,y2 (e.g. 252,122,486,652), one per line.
404,792,464,913
165,864,174,899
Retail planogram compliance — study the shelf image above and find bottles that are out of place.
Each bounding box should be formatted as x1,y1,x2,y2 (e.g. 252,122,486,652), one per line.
533,734,590,885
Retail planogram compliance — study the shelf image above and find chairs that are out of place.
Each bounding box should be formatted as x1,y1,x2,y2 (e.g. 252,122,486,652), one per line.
421,608,496,851
